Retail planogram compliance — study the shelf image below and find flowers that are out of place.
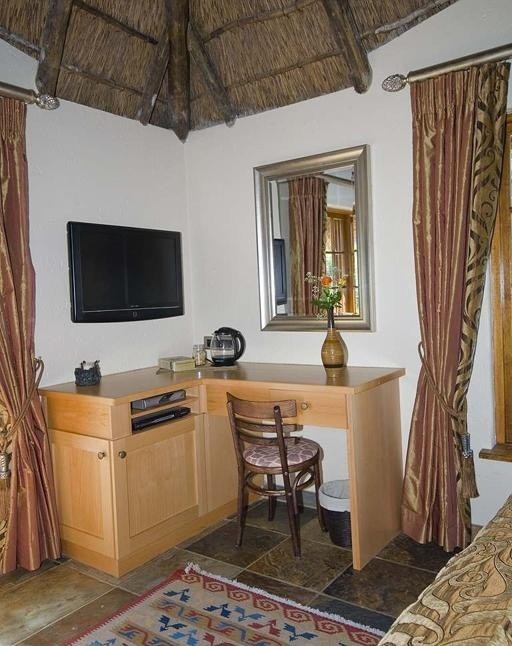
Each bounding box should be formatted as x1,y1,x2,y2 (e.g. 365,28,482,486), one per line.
304,267,347,326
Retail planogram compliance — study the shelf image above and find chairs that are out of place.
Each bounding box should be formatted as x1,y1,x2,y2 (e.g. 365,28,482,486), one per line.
226,392,328,558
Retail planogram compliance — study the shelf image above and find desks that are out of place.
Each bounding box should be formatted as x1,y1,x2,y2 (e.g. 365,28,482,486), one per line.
34,362,406,579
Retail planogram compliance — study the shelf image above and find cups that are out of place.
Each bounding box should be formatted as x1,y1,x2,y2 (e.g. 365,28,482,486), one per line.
192,344,207,366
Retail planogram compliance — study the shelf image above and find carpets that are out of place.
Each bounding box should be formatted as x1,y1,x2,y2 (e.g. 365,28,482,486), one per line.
61,562,385,646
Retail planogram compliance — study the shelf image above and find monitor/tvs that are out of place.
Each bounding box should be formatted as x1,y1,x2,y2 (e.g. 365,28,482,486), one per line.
67,221,185,323
273,238,288,305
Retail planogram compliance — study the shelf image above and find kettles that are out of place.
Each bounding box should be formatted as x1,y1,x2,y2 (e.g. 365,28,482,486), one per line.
210,327,246,366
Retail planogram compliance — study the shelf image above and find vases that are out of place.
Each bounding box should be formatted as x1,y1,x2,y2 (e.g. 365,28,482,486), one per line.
321,328,349,378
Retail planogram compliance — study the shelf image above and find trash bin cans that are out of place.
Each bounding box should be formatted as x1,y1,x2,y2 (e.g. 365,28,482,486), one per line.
318,479,352,547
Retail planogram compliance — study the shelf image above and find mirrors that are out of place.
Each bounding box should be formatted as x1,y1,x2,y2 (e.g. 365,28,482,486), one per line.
252,144,373,332
